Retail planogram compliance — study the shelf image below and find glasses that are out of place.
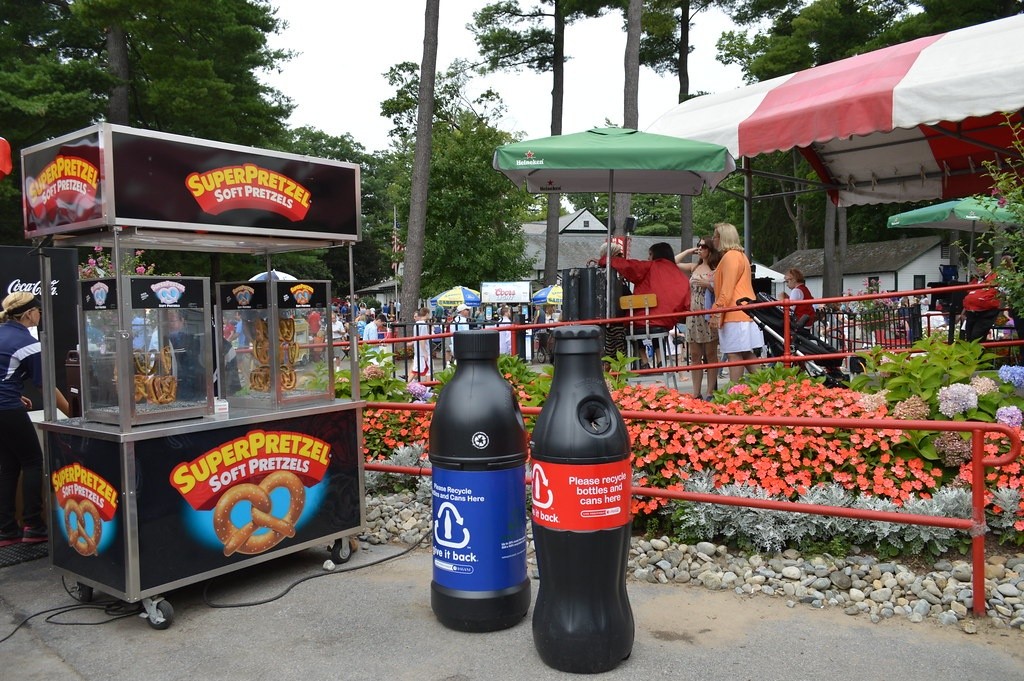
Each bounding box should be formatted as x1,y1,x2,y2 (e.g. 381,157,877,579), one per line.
697,243,709,249
711,235,720,238
783,278,792,284
615,253,623,258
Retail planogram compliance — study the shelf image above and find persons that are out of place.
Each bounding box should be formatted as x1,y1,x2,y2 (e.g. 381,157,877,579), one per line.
964,255,1024,344
587,221,815,402
814,291,966,345
223,311,249,348
170,308,243,401
305,299,562,382
0,291,69,545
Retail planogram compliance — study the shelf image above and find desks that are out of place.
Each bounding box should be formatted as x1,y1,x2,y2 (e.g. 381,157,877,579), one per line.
991,326,1019,368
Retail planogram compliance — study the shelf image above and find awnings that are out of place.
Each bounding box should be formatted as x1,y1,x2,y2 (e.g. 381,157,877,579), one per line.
644,13,1024,261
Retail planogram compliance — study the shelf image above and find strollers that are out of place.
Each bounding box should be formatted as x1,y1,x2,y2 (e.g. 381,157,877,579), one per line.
736,291,867,390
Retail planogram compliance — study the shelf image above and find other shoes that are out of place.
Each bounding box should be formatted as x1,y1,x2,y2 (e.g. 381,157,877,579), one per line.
706,395,715,402
722,371,727,375
719,375,725,379
680,377,689,382
633,363,650,371
695,394,703,400
336,367,340,373
658,361,662,368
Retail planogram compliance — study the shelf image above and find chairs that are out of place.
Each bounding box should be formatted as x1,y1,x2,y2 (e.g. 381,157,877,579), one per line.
619,294,677,389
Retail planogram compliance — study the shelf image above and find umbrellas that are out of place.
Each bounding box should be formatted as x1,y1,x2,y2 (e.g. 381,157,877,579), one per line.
249,268,298,280
887,195,1024,284
532,285,563,319
430,286,480,306
491,126,736,327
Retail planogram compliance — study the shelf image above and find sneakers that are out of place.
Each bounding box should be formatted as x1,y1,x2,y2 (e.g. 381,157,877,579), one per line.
22,523,48,542
1,523,24,546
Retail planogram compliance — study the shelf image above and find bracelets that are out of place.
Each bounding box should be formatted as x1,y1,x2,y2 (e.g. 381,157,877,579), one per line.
713,313,720,318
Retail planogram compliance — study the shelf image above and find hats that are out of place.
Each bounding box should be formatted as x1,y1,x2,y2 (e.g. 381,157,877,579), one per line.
456,304,471,312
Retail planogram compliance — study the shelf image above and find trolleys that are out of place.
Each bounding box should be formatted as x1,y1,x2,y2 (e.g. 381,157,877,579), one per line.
19,113,366,631
530,285,564,364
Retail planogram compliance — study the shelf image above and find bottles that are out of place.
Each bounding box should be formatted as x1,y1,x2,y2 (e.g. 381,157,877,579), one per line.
427,330,531,633
529,325,635,674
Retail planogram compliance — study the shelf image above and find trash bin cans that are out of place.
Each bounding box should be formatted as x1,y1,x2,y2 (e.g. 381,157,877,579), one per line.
429,331,531,635
529,326,634,673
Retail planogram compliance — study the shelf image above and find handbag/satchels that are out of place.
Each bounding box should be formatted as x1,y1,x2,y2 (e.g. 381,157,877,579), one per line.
672,335,686,347
